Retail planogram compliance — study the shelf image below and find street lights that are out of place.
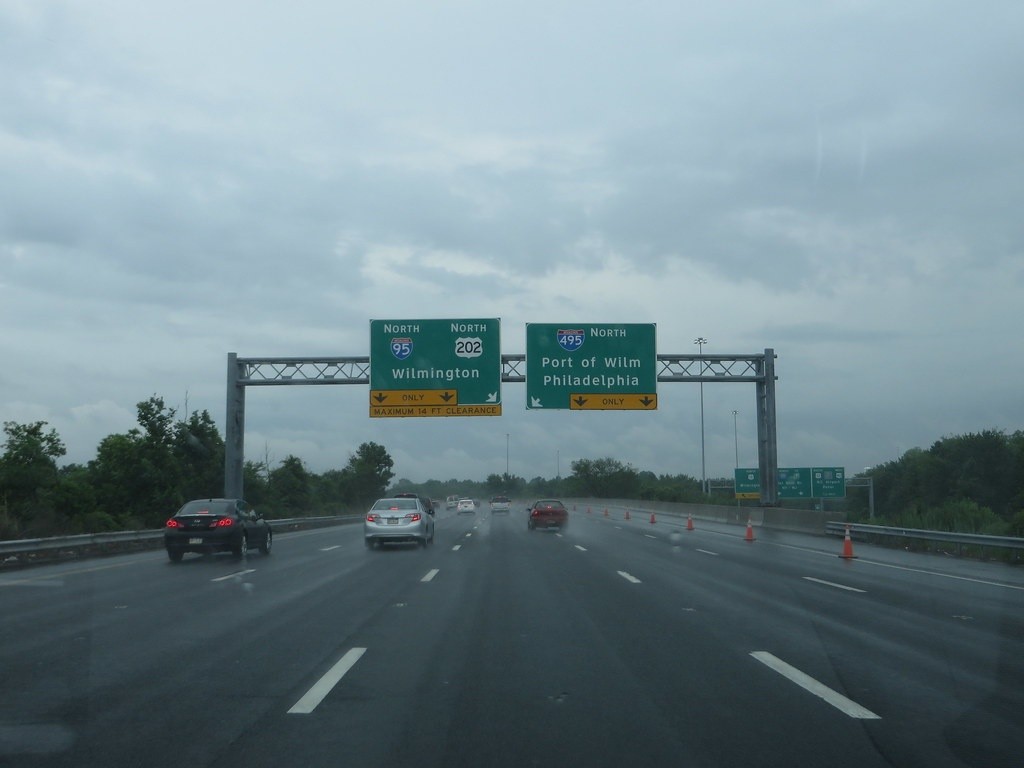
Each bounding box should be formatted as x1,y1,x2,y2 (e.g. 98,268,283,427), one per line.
693,337,708,491
504,433,512,476
733,410,740,468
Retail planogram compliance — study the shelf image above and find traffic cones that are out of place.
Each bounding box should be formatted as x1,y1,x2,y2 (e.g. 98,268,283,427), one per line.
624,510,631,520
837,524,861,560
573,505,577,511
742,523,754,540
586,506,592,513
602,508,610,516
685,518,695,530
648,513,656,523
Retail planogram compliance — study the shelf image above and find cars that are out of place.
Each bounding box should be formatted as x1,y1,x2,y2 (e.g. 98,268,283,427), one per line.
490,497,512,515
164,497,272,561
365,498,435,549
394,493,417,498
432,501,440,509
526,500,570,532
457,500,476,516
446,496,458,510
419,497,434,515
